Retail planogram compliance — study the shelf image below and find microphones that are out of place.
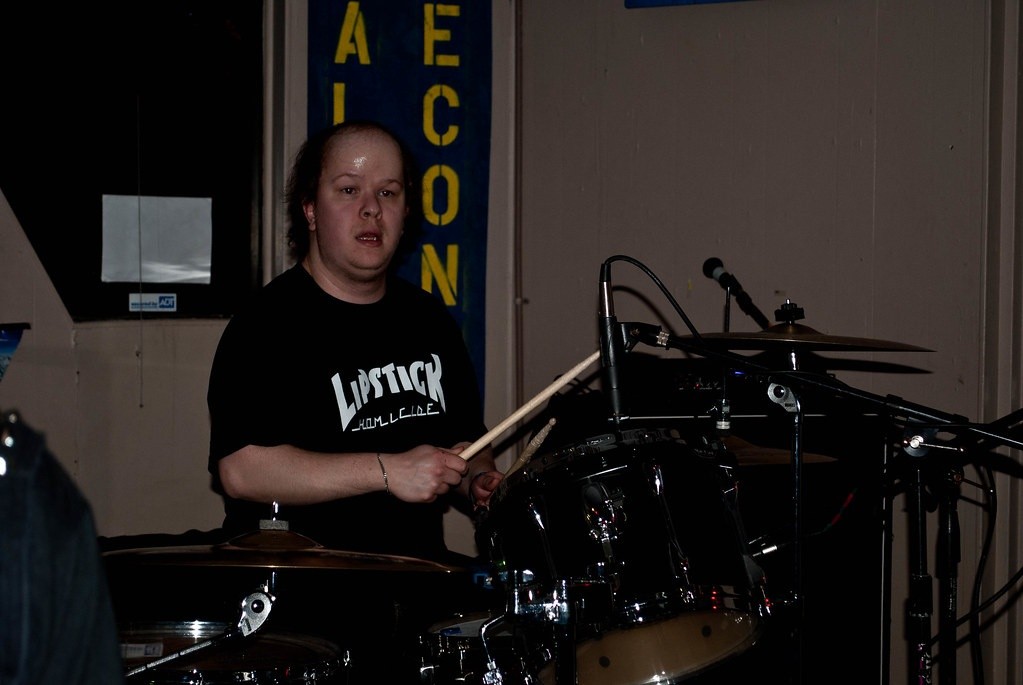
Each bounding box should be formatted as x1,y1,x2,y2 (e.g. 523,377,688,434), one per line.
703,257,734,290
597,262,623,421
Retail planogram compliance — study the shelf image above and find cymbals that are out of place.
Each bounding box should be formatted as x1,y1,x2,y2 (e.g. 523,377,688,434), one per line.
661,322,941,354
100,523,460,576
717,432,839,468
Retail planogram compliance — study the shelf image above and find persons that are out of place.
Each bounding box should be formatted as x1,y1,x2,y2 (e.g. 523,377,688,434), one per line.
206,119,505,551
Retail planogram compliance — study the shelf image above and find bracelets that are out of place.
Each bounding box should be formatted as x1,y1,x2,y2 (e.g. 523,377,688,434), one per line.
378,453,388,492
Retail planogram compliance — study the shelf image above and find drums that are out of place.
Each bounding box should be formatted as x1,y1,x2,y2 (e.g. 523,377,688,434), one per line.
121,616,364,684
483,430,787,684
425,611,560,684
